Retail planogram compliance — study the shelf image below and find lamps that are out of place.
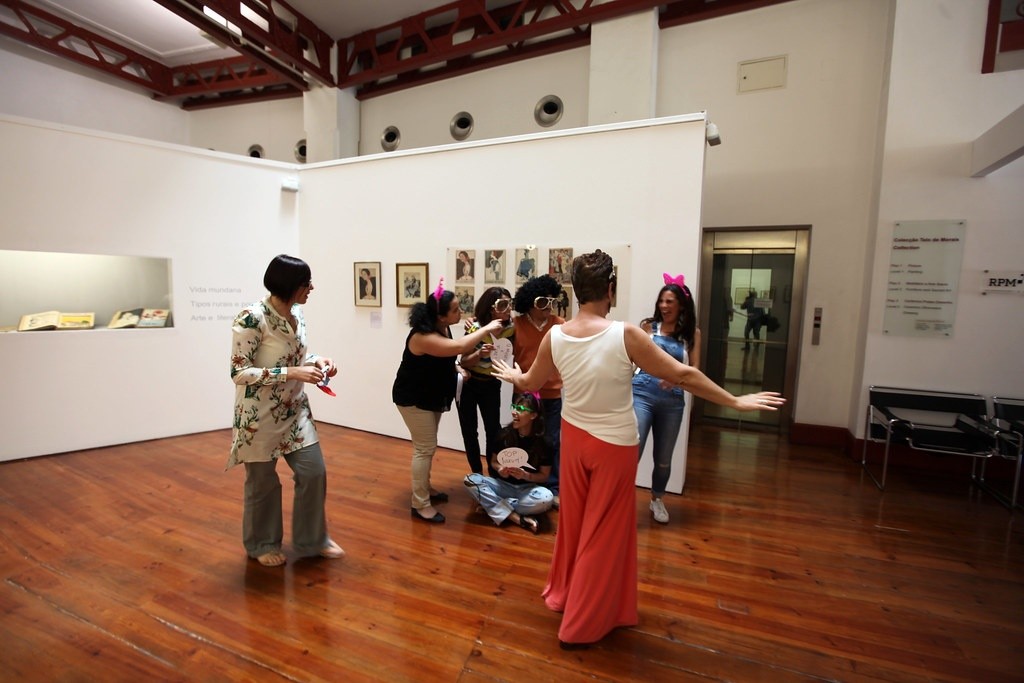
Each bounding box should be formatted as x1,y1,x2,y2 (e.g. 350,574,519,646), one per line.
706,119,721,147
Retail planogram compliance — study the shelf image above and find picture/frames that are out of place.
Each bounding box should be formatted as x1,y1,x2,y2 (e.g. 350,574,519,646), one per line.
396,262,430,308
353,261,382,307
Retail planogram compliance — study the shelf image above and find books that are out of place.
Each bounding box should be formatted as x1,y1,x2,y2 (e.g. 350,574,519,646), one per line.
18,311,95,331
107,307,170,328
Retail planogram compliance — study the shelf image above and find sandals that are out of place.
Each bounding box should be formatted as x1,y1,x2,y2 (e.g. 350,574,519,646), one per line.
320,539,344,559
257,553,285,566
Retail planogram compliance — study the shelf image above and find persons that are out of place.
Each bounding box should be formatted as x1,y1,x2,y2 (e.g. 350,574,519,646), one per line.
740,289,765,351
491,252,787,643
464,273,566,509
230,254,345,566
463,391,553,535
631,272,701,523
392,276,503,522
354,247,573,324
456,287,513,481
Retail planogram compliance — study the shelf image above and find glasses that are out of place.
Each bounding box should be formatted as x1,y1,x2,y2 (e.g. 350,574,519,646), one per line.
492,298,514,313
534,297,559,311
510,403,534,414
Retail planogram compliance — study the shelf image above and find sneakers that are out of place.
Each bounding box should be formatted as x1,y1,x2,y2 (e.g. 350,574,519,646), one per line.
650,498,671,523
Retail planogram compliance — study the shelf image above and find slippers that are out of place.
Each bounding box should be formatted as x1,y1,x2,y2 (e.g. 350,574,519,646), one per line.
519,514,540,534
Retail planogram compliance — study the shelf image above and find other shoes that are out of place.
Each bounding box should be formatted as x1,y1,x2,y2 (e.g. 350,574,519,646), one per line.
411,507,445,523
430,492,448,501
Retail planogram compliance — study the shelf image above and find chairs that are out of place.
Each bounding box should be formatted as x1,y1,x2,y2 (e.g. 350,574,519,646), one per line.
861,385,1024,513
